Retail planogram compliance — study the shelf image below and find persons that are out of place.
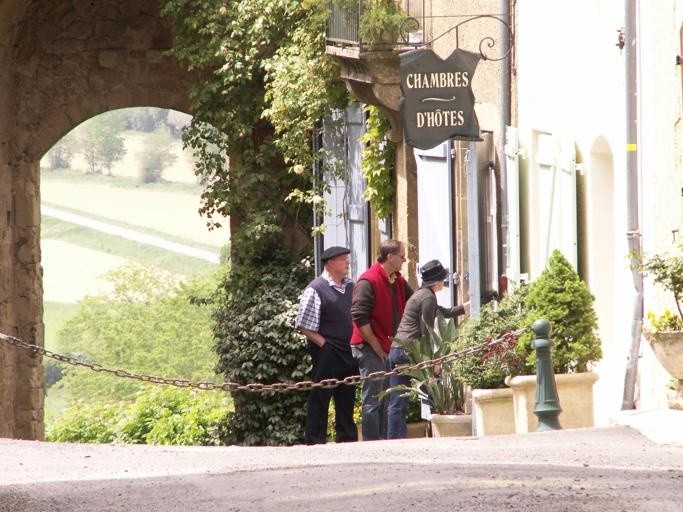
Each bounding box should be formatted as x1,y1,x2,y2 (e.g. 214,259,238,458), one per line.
349,239,415,440
387,260,470,439
294,246,358,445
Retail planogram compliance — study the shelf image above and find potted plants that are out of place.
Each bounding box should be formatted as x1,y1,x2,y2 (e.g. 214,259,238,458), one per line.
366,307,471,438
628,240,683,408
511,248,604,433
451,289,518,439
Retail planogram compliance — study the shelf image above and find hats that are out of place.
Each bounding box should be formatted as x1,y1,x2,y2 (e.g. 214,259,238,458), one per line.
417,258,451,287
319,246,351,262
